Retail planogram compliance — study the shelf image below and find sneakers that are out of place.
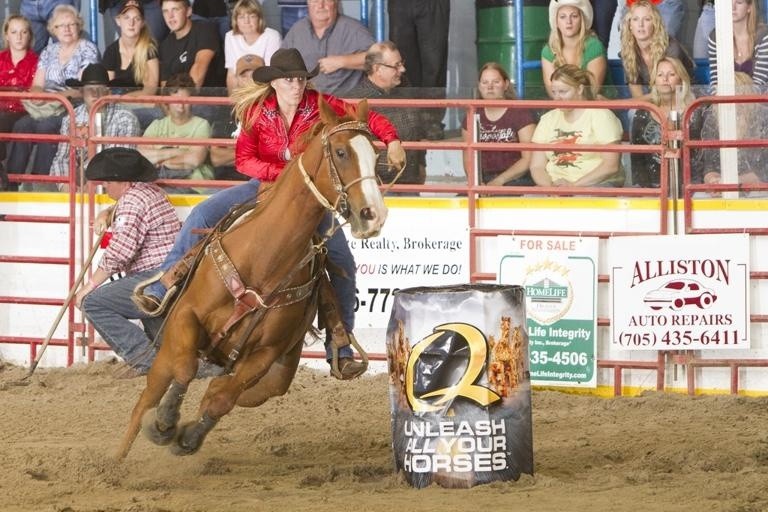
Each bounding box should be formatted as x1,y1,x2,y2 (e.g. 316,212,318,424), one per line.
112,363,143,379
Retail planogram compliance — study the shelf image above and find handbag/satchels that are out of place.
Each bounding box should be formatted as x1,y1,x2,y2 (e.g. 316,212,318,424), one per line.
21,80,75,121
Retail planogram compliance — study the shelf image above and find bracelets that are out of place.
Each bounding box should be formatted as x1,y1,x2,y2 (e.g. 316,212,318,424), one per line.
88,279,99,289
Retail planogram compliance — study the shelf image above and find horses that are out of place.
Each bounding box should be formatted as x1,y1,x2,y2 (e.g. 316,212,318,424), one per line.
114,87,389,465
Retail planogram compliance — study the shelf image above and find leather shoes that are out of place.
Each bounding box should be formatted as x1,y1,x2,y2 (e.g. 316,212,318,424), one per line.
329,357,364,377
130,292,160,313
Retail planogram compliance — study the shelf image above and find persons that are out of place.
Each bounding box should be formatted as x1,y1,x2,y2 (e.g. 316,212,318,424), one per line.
130,46,406,379
73,147,230,379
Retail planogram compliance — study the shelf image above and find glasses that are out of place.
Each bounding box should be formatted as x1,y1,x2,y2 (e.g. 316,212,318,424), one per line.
378,56,406,70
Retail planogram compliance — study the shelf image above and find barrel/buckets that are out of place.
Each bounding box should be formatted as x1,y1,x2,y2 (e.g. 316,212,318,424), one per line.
385,284,534,488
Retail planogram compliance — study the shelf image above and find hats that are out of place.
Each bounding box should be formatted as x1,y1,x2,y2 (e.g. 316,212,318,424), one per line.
80,64,109,86
252,48,319,83
548,0,594,32
236,54,265,78
120,1,145,17
86,147,159,182
